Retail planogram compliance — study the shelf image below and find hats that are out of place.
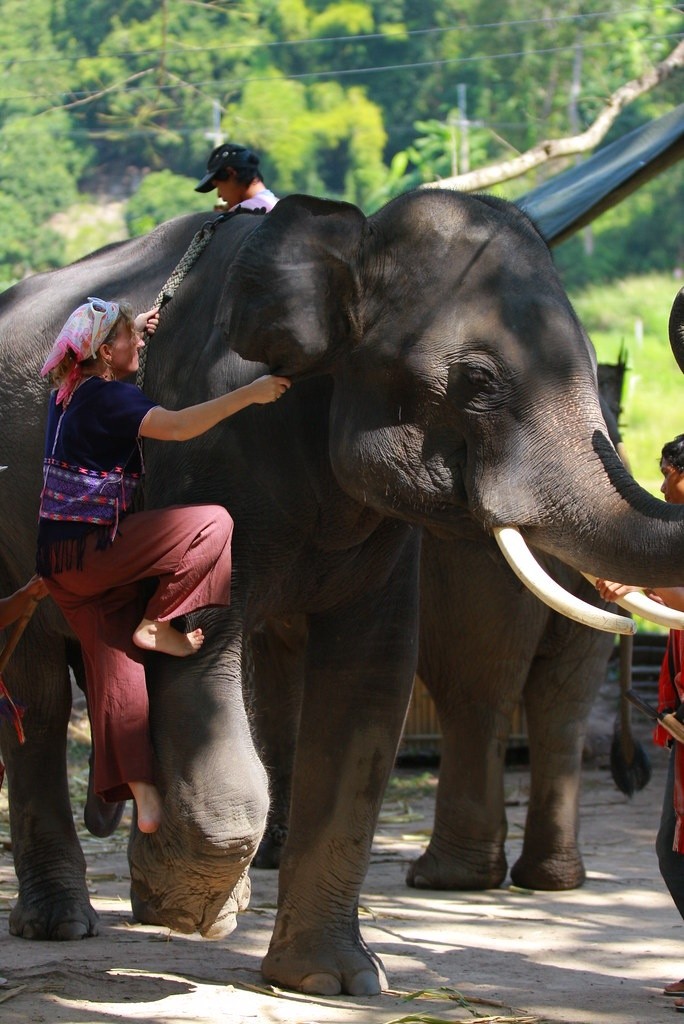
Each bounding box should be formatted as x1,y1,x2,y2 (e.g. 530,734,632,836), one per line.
195,143,251,193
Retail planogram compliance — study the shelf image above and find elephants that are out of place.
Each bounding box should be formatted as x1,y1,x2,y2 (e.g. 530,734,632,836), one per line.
1,188,683,1003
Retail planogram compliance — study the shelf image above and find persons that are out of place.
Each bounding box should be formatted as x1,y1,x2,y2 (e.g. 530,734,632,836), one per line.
595,434,684,1009
0,573,51,1005
193,142,281,213
36,299,292,834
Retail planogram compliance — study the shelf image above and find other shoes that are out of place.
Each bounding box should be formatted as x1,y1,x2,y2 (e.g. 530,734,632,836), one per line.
664,979,684,1013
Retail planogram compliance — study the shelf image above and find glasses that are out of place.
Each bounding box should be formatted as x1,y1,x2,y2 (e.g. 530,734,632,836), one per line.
87,297,109,359
